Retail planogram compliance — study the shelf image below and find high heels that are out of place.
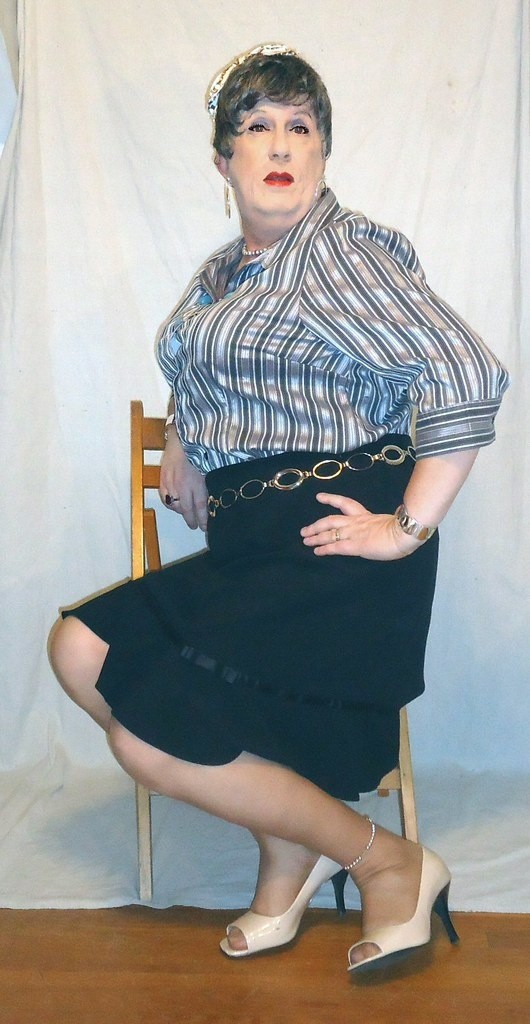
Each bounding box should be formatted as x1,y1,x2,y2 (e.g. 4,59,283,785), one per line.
347,843,459,983
219,854,348,959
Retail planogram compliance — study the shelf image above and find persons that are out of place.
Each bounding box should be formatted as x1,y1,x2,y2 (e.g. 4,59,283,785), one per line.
50,44,512,974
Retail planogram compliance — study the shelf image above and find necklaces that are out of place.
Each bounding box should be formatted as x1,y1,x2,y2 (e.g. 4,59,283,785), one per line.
242,243,266,256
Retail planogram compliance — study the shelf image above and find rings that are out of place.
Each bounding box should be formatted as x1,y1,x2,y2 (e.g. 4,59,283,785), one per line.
335,531,340,541
165,494,179,505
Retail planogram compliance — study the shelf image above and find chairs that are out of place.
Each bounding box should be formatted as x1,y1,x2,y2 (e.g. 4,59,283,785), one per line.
131,401,417,902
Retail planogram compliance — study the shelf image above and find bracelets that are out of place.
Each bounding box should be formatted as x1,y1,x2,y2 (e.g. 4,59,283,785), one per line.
395,504,436,541
163,414,175,441
